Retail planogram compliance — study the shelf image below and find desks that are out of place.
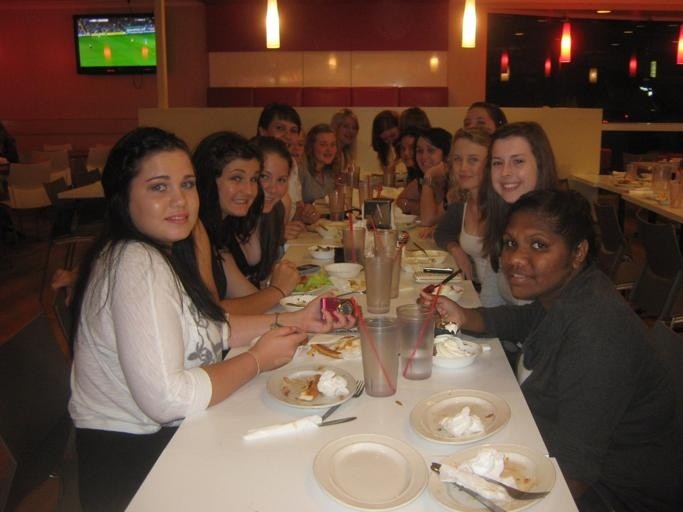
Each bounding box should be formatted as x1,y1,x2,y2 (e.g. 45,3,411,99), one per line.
122,180,580,511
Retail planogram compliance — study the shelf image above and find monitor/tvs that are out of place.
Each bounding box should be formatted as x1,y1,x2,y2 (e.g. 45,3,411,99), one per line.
73,12,157,76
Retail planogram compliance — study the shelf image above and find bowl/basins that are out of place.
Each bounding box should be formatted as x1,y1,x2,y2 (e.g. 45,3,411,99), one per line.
326,262,364,281
430,337,481,370
411,249,449,265
314,226,338,240
306,244,336,263
428,285,466,302
274,293,315,313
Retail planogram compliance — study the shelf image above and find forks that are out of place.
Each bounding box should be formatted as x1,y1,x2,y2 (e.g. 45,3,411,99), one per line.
319,378,364,421
435,459,551,501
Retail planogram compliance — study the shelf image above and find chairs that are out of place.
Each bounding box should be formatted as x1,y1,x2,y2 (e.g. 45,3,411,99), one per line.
0,143,104,512
562,177,683,408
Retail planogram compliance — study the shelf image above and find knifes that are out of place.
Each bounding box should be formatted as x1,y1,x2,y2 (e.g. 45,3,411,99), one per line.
319,415,359,430
447,480,505,512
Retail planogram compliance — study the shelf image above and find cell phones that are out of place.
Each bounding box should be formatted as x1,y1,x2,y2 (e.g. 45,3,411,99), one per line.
296,264,321,274
423,267,453,273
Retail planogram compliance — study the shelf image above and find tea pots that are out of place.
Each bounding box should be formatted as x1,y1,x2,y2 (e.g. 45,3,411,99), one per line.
650,161,683,207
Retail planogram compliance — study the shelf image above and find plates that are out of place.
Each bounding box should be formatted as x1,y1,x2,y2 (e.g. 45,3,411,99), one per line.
409,388,511,443
430,444,559,512
264,364,354,410
309,435,429,510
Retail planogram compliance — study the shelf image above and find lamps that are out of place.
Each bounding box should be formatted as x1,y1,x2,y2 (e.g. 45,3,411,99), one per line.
266,2,280,50
462,1,478,48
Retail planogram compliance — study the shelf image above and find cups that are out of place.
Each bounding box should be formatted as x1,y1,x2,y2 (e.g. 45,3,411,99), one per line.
328,159,383,222
373,231,399,255
395,303,440,381
333,246,361,263
627,163,638,180
385,172,397,189
342,226,368,267
364,255,391,315
360,318,403,398
371,244,402,297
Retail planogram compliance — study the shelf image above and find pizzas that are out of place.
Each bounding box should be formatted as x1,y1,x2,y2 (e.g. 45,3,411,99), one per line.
298,374,322,400
312,344,344,357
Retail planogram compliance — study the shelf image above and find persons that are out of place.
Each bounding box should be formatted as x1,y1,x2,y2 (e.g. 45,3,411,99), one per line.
246,98,559,388
62,124,361,511
0,122,18,172
413,188,682,512
172,128,303,316
